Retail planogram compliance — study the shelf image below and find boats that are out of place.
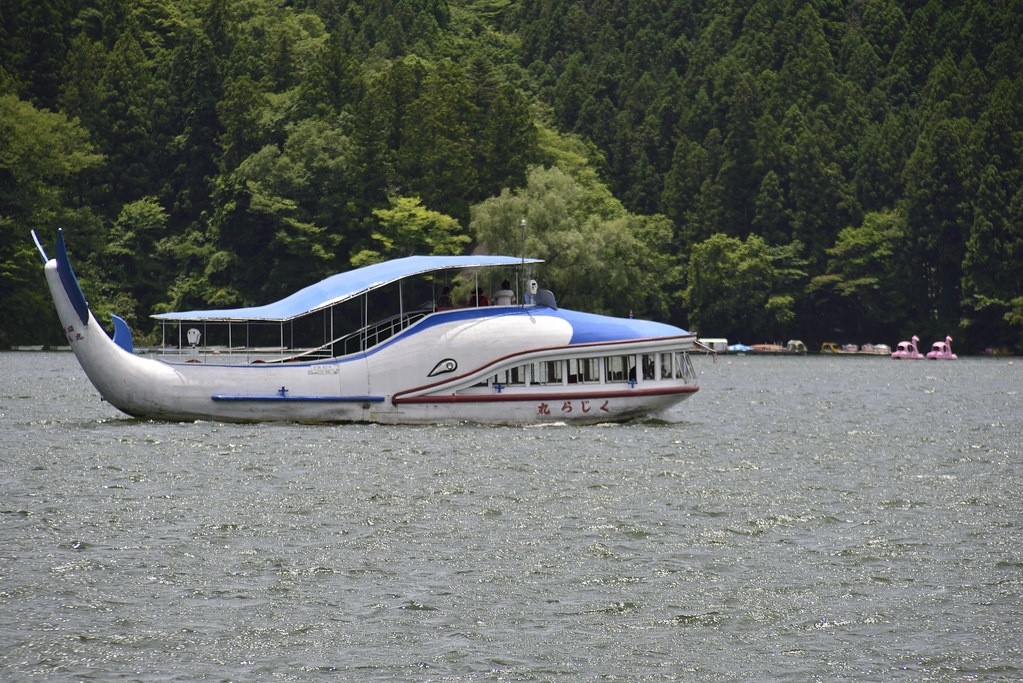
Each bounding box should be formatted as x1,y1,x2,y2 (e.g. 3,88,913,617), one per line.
926,336,958,361
30,226,699,425
819,342,890,356
749,339,809,355
891,335,925,360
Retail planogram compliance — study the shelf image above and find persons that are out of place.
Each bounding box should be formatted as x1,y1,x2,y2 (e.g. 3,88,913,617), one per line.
469,287,489,307
494,279,516,306
437,287,453,306
